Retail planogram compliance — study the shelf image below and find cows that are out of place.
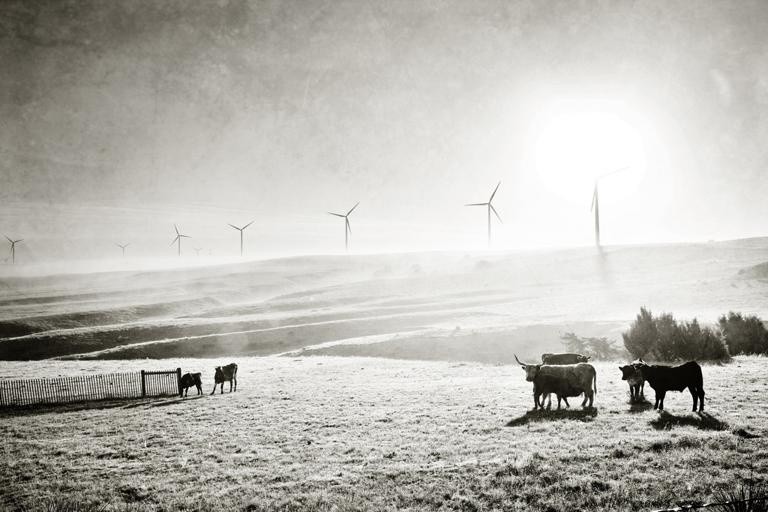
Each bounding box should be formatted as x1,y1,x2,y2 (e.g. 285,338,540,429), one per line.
209,363,239,395
636,361,705,412
618,358,647,403
179,372,203,396
513,352,597,410
